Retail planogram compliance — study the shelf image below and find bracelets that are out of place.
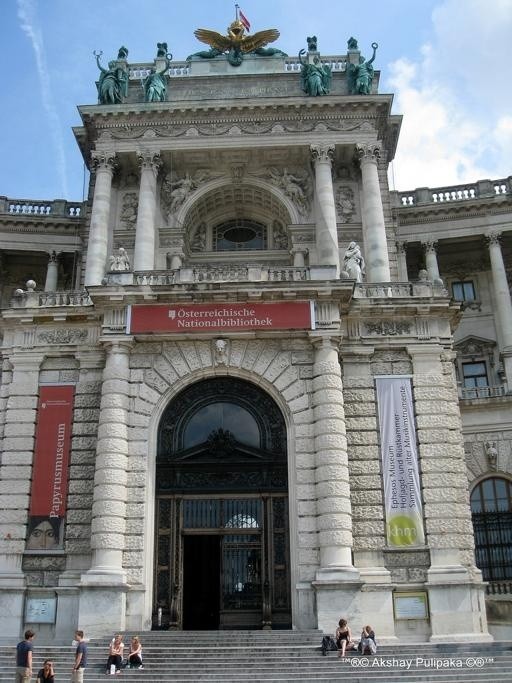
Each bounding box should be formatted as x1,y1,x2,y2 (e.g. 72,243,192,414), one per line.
73,666,77,669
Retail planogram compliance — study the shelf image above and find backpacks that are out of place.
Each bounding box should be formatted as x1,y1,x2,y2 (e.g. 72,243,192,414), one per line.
321,636,339,655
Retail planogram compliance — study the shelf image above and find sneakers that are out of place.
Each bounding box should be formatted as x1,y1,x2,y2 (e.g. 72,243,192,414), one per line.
104,669,121,676
127,663,145,670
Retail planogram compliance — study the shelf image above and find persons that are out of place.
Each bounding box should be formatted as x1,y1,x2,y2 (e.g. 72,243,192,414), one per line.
348,42,379,95
272,167,307,202
297,48,328,96
336,618,354,658
110,247,131,272
360,624,377,656
36,659,57,683
24,514,60,548
68,630,88,683
141,58,170,101
105,638,123,674
92,55,126,105
15,630,35,683
343,241,366,283
111,633,124,660
166,169,197,214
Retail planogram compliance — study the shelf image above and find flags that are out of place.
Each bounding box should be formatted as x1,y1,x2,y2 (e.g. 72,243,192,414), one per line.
240,11,251,31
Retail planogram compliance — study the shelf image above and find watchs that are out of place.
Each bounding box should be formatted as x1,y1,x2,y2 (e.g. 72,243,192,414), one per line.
126,635,144,670
